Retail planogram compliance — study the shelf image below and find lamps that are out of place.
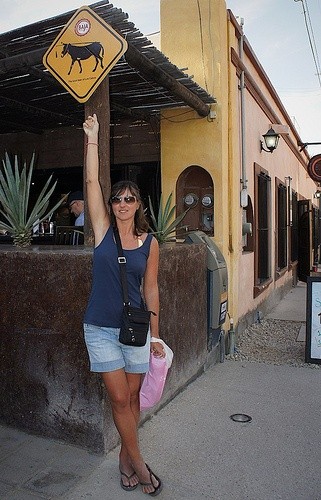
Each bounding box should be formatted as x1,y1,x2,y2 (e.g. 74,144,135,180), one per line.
259,125,280,153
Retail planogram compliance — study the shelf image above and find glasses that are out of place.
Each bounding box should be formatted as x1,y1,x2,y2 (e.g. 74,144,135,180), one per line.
110,195,138,205
67,202,75,210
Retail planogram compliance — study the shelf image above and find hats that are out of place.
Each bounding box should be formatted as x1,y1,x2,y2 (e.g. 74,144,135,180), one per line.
61,190,84,209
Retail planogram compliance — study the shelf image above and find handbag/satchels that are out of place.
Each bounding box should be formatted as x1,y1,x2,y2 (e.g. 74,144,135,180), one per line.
119,303,158,348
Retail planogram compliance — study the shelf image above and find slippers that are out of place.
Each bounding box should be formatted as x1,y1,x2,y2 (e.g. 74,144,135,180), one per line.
138,461,163,497
119,469,138,491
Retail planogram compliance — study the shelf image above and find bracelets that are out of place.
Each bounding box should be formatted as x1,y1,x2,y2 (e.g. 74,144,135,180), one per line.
85,142,100,147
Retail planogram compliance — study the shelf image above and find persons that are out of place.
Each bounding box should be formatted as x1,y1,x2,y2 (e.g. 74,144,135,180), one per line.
66,191,85,226
83,113,167,498
